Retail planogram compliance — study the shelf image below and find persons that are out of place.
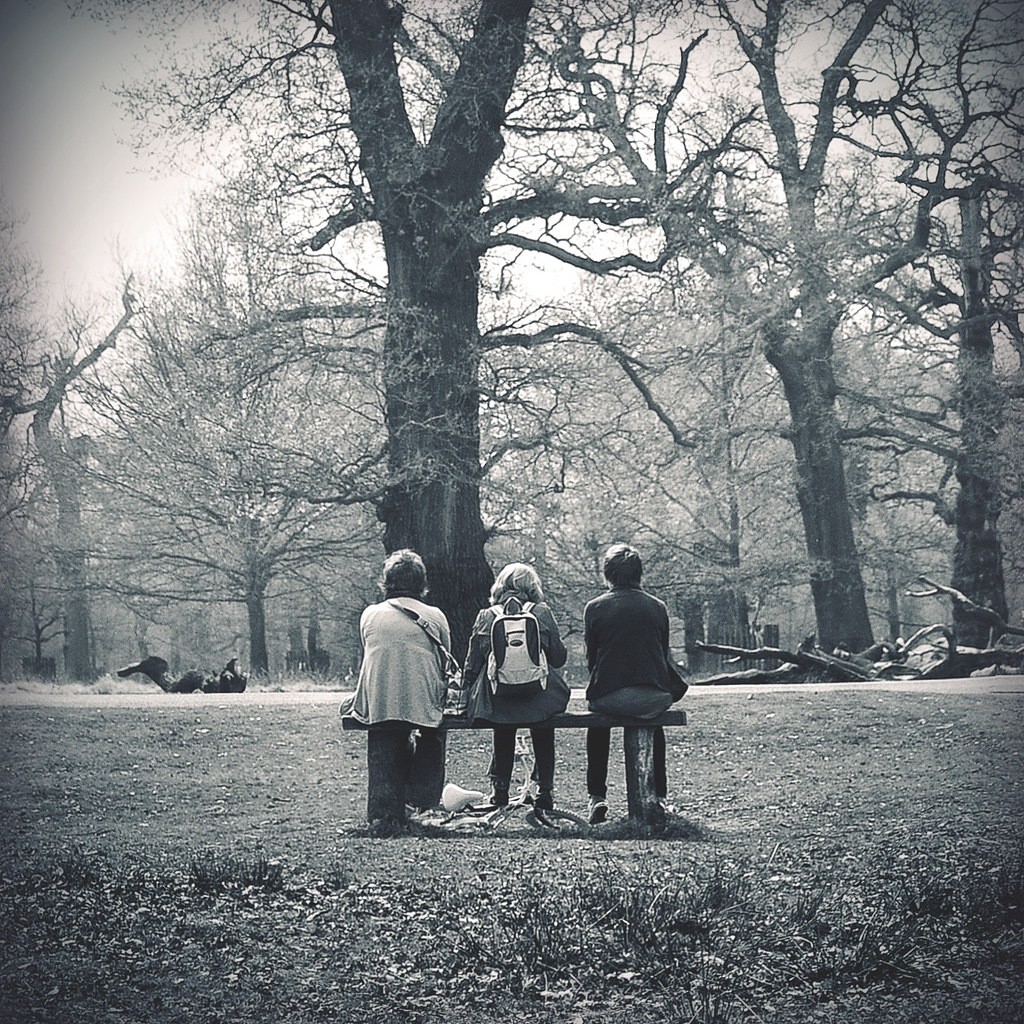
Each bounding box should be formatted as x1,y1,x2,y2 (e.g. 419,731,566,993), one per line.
583,543,689,825
340,548,452,809
463,562,571,809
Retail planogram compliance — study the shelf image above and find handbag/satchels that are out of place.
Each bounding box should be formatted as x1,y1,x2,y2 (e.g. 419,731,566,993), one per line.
442,654,469,717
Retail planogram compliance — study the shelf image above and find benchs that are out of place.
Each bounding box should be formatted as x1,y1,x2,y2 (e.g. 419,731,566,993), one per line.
342,710,687,829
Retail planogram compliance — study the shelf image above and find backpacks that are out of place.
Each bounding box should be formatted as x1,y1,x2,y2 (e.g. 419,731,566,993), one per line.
487,596,548,696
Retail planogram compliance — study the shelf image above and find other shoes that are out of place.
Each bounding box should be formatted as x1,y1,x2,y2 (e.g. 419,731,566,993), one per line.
587,794,608,823
533,785,553,809
489,787,509,806
